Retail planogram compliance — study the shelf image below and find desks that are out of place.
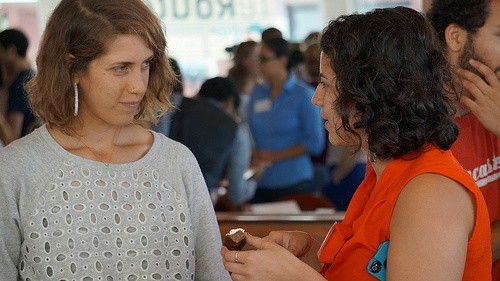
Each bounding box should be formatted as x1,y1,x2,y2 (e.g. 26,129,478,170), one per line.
215,211,345,273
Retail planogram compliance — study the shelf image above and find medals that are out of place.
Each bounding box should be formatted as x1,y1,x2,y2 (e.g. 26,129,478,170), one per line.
153,27,370,221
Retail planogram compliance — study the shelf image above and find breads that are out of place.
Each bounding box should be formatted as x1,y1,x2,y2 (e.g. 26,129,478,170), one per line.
223,228,248,251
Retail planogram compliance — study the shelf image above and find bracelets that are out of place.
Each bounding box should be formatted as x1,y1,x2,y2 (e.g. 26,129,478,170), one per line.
297,230,313,257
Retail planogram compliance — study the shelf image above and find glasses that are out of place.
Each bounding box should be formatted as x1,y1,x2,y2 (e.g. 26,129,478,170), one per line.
253,56,278,63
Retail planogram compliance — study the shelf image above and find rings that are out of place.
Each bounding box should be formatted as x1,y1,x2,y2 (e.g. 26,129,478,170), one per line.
234,250,240,264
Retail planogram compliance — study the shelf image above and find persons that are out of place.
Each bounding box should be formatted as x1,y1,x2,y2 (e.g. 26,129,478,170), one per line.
1,0,235,281
220,6,491,280
425,0,500,281
0,24,43,149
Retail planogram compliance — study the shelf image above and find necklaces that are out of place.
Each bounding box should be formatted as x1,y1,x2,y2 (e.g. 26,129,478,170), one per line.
68,124,123,170
